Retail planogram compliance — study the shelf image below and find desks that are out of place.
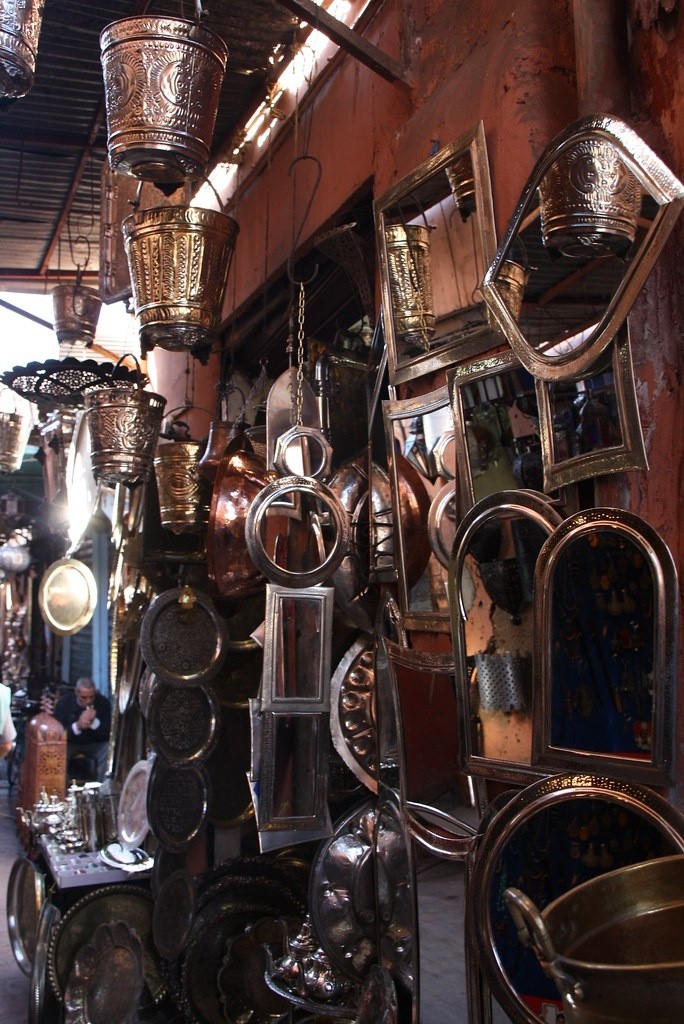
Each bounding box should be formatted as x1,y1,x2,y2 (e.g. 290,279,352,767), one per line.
41,833,155,912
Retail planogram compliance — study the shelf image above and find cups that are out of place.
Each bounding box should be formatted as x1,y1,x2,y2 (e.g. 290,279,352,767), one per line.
79,794,120,852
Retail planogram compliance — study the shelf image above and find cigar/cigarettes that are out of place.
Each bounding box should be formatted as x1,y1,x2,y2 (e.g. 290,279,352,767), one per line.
86,705,89,710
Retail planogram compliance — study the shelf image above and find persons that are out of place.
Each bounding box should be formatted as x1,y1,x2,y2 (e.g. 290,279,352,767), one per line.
55,676,111,786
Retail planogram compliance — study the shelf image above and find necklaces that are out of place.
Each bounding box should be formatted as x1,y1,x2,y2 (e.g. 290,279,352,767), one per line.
0,682,18,761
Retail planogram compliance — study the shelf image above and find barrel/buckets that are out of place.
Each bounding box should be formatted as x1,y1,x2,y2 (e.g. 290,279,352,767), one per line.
155,442,212,527
118,177,240,352
0,0,45,97
443,154,476,226
100,0,228,187
539,140,644,261
85,352,168,487
50,284,104,344
380,203,438,338
0,387,35,474
496,259,537,325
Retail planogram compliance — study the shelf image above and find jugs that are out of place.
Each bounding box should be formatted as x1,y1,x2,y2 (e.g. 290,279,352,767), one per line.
260,913,352,1006
15,779,84,837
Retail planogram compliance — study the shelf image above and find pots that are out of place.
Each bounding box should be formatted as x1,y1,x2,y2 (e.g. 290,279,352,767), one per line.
504,854,684,1024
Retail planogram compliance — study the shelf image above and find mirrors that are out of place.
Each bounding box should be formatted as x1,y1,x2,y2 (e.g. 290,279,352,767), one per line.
529,315,649,494
479,113,684,383
245,477,350,588
372,122,508,388
445,348,567,511
403,812,483,1024
262,583,334,712
260,712,330,833
381,636,489,860
275,425,333,478
380,385,463,636
